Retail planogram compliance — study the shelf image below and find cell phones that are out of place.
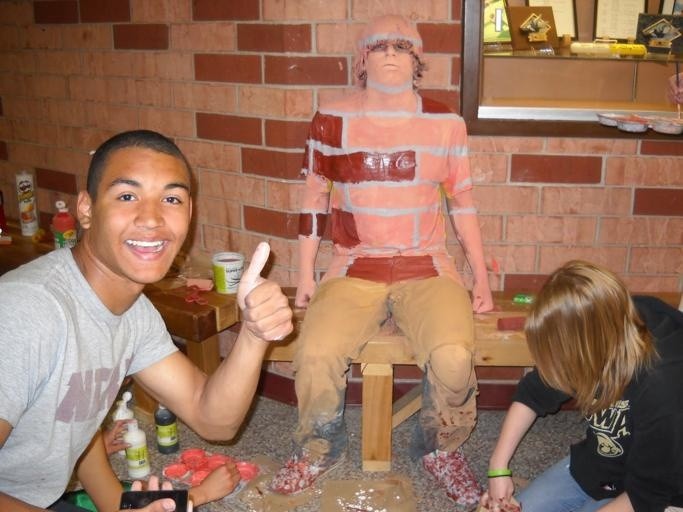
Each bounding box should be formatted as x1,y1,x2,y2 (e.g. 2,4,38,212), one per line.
120,490,188,512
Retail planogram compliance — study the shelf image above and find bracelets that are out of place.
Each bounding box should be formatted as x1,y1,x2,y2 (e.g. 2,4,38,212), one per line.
487,469,513,477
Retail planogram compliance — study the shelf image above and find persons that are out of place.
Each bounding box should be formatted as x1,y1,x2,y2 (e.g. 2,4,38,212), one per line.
271,17,496,510
486,258,682,512
76,418,241,511
0,131,295,512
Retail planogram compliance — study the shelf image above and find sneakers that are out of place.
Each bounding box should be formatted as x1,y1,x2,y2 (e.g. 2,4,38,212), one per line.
266,433,351,499
418,442,484,509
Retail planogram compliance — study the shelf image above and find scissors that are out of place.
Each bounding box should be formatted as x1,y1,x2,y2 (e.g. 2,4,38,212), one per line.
185,288,208,305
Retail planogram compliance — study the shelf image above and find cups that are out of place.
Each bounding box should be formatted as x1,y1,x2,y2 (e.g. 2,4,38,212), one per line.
210,251,244,294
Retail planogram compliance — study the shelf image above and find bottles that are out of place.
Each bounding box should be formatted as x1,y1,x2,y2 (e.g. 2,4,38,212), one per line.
111,389,180,479
51,201,77,251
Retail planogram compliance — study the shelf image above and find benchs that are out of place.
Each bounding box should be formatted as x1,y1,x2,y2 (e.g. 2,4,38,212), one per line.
258,289,543,476
0,223,243,421
